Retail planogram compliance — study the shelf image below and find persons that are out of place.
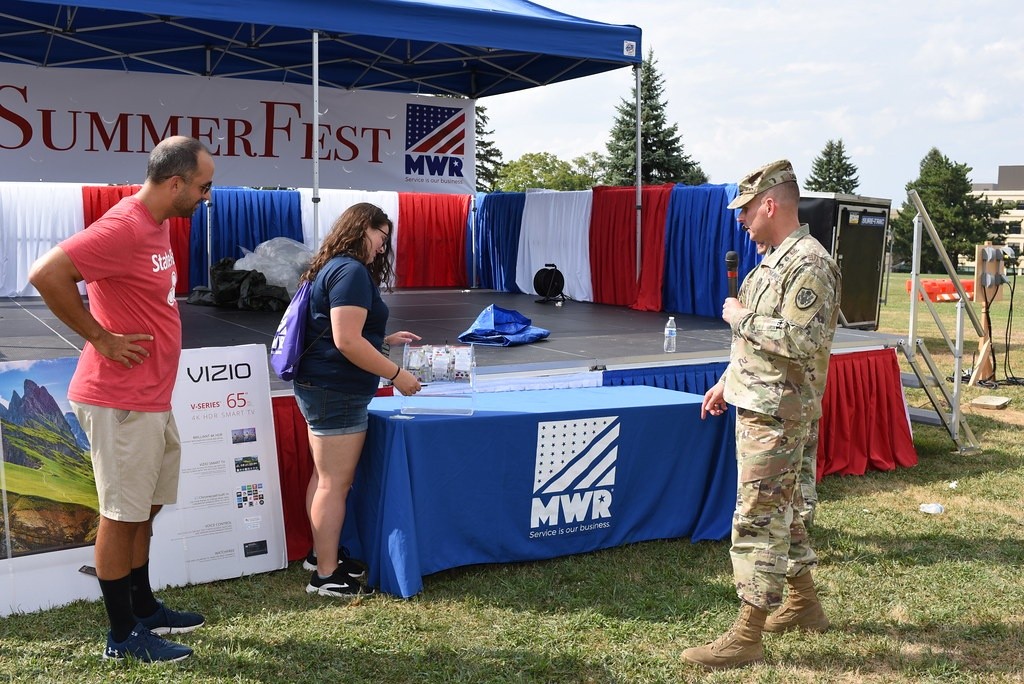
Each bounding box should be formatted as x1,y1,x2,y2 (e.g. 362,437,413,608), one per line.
681,159,842,667
294,203,423,598
28,136,214,666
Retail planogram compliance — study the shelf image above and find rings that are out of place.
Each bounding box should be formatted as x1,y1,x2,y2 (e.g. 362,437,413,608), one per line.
714,404,719,406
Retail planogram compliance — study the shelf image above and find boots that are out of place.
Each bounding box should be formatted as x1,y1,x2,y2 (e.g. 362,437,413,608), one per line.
762,570,829,633
679,601,768,670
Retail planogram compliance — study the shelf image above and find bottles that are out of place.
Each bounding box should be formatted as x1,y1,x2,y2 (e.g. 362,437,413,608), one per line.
920,502,944,514
664,317,676,352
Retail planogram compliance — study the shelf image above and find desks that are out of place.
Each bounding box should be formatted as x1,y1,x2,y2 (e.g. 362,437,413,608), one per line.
342,385,737,599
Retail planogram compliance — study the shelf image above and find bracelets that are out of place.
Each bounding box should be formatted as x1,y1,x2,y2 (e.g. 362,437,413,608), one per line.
390,367,400,381
384,337,387,344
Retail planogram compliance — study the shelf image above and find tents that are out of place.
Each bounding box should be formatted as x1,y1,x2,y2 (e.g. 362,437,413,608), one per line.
1,1,643,284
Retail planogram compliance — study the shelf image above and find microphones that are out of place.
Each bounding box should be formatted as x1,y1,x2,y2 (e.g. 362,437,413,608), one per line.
724,251,738,301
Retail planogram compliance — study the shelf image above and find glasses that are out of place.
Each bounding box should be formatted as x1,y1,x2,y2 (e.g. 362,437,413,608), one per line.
179,176,213,195
374,227,390,246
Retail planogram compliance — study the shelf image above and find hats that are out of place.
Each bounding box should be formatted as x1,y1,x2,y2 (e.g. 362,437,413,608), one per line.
726,159,798,210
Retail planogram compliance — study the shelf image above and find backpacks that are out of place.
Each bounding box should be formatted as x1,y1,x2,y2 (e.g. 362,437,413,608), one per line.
269,274,330,382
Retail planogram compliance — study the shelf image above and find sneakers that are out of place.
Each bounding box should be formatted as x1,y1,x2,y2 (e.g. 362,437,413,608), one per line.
304,567,375,598
131,597,206,637
302,546,366,578
102,622,194,666
